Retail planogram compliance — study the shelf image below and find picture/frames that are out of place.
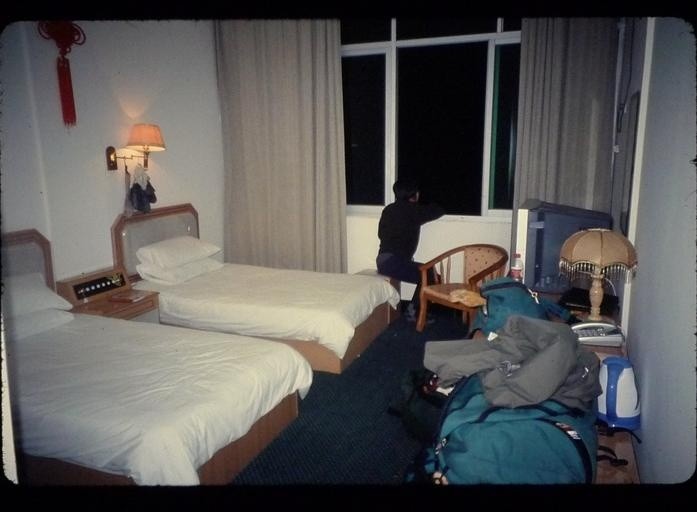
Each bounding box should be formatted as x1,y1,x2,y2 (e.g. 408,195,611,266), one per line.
619,88,640,237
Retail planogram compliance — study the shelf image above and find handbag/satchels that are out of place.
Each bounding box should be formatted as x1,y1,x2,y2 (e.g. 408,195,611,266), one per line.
423,372,641,485
470,277,584,338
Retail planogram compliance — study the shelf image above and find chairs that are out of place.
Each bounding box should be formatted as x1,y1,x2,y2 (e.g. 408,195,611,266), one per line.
415,243,509,338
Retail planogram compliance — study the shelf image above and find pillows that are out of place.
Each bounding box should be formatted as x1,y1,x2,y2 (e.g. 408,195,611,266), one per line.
1,273,73,312
136,258,228,281
5,310,74,340
136,236,224,271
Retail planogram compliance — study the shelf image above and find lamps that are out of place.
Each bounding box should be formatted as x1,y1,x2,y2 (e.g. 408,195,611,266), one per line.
558,228,640,321
106,123,167,171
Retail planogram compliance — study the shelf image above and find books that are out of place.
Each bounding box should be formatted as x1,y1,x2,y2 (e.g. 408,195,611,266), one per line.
109,291,147,303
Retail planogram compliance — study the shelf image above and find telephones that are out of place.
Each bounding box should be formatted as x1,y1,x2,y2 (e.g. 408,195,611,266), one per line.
570,321,623,347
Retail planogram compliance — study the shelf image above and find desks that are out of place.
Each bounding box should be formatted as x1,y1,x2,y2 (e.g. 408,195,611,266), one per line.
64,289,160,323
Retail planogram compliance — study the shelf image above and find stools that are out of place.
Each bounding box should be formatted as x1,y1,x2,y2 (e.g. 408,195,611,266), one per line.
353,268,401,322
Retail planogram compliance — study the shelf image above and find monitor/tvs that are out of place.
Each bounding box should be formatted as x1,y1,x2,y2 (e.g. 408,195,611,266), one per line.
508,198,614,295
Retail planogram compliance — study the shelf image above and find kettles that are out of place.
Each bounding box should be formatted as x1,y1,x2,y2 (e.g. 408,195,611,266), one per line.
596,356,641,432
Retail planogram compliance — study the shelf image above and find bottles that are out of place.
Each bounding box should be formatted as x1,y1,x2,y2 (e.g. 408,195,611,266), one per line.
511,253,524,284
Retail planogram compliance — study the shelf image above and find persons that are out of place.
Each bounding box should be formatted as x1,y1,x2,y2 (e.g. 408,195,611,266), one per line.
376,180,444,294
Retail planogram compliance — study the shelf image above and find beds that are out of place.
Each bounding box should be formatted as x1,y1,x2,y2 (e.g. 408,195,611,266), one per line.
0,312,314,486
131,263,401,378
